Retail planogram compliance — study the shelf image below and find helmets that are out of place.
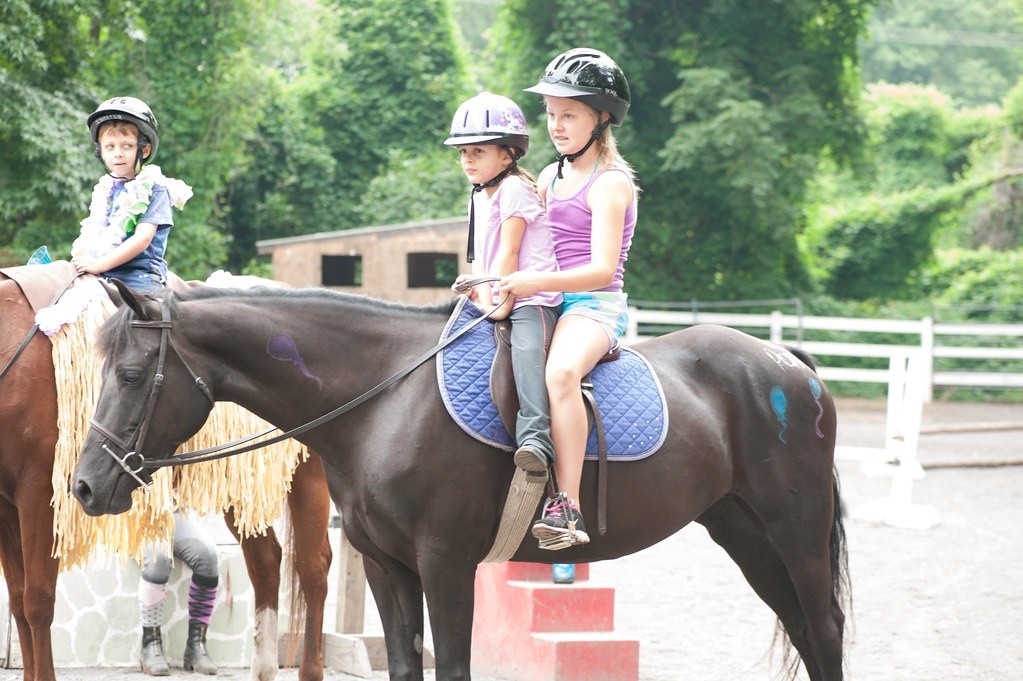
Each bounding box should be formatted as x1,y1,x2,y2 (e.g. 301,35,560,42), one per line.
87,96,159,166
523,48,631,128
444,92,529,158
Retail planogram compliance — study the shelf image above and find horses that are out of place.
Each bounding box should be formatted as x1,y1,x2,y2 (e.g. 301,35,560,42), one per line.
0,261,853,681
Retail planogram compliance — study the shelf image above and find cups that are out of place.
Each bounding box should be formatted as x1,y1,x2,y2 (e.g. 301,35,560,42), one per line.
27,246,51,266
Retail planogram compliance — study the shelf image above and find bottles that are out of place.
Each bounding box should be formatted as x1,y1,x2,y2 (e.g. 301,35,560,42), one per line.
552,564,574,583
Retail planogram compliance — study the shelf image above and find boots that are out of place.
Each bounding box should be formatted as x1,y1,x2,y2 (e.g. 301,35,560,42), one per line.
140,626,170,676
184,624,218,676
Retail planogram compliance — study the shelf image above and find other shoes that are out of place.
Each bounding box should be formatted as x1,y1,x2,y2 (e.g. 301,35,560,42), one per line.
514,444,549,483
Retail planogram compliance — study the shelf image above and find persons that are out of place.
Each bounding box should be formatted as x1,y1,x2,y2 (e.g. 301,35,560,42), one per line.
443,46,642,545
127,499,220,677
72,94,174,292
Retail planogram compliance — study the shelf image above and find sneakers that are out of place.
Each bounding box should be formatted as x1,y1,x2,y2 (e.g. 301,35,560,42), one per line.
531,499,590,545
